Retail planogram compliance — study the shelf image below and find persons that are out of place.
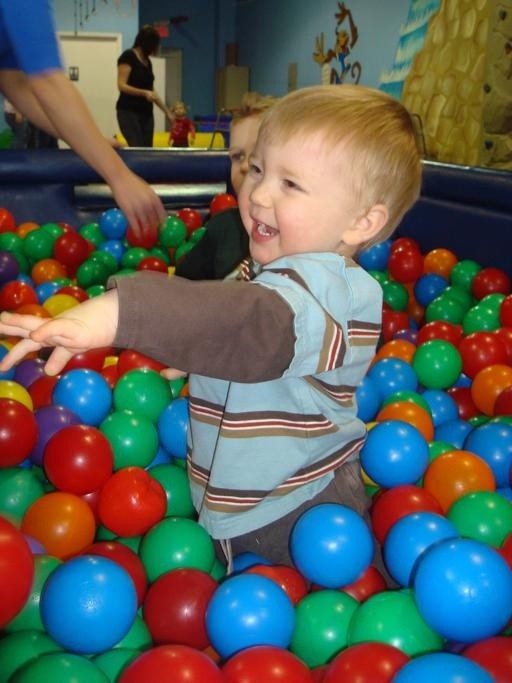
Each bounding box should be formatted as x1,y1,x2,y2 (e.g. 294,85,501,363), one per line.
116,25,171,146
161,93,288,379
168,100,195,147
0,1,166,242
4,98,58,149
0,84,424,590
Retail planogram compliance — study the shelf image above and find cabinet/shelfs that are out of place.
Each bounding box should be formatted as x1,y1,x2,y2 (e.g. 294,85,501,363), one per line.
215,65,249,114
